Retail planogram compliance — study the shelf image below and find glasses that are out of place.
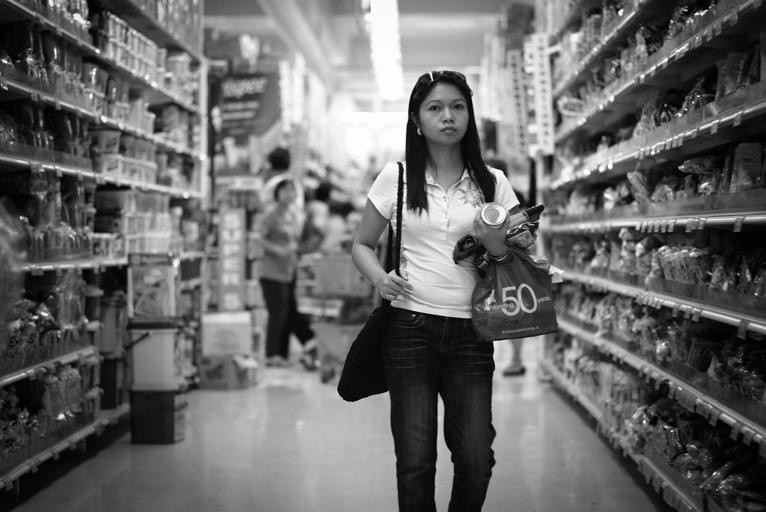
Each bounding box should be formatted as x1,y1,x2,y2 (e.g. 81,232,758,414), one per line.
407,71,465,114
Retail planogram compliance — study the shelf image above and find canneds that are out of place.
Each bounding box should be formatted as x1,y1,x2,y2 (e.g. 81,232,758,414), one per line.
481,203,506,229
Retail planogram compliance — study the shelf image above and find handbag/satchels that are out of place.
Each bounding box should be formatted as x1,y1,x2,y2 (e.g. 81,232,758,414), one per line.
337,306,389,402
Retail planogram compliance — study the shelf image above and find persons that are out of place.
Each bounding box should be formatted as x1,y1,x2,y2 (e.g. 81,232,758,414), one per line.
350,67,531,512
253,147,356,371
486,159,527,376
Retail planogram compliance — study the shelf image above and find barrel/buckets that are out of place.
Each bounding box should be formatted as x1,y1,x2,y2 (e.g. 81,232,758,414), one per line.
124,321,196,383
125,384,191,444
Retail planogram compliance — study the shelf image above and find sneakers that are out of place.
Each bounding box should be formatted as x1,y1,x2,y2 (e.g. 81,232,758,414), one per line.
265,355,290,367
302,337,319,364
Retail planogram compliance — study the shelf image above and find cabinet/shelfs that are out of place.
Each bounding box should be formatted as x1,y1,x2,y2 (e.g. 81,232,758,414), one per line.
500,0,766,512
1,1,356,511
